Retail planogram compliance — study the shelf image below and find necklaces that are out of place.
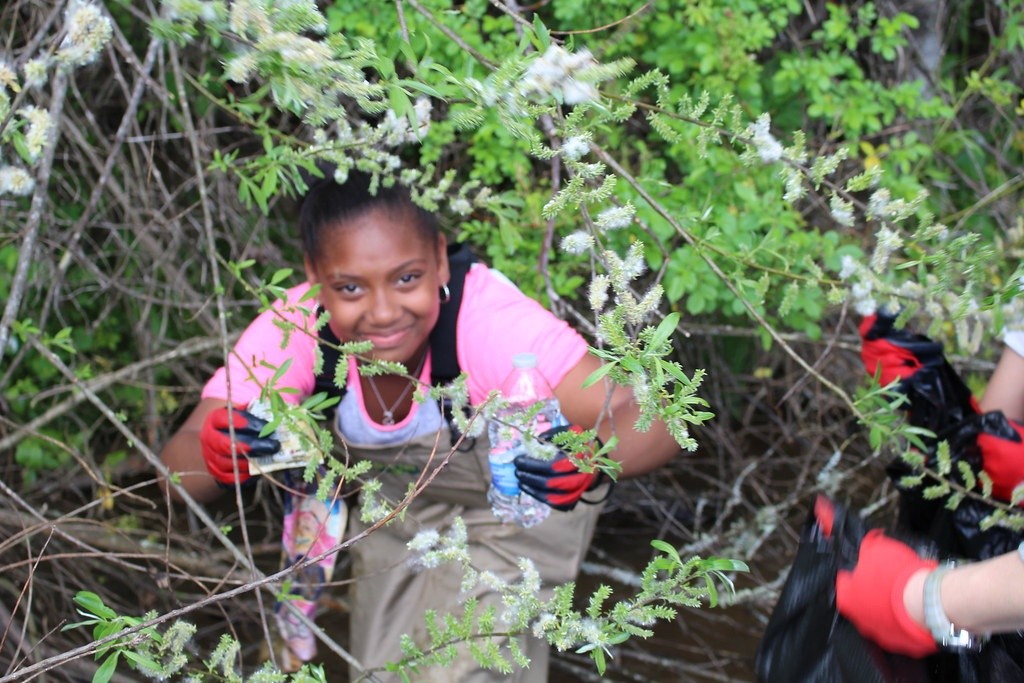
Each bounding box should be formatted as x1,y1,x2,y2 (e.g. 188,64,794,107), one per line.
367,357,424,424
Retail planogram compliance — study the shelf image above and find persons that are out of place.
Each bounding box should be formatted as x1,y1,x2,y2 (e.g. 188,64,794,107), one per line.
155,176,687,683
754,264,1024,683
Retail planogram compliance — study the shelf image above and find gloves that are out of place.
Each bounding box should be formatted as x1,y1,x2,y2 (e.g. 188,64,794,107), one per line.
965,394,1024,508
514,423,607,511
200,403,279,491
859,310,946,391
813,489,940,659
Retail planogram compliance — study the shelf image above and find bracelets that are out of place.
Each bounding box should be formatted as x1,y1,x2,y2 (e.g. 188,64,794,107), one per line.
922,557,987,652
